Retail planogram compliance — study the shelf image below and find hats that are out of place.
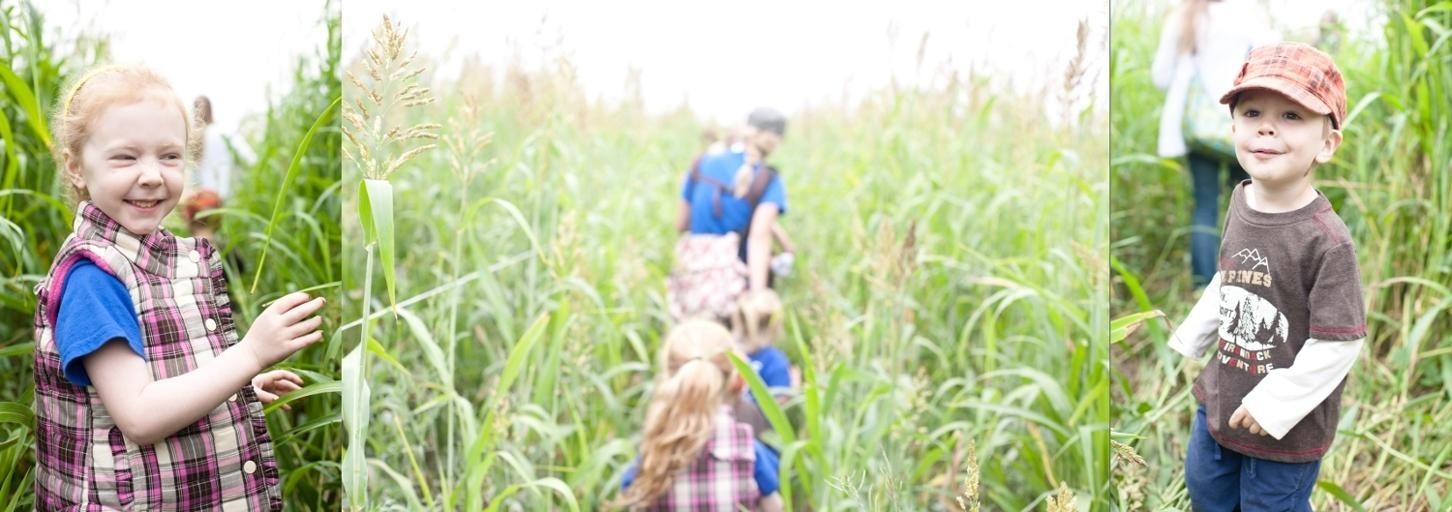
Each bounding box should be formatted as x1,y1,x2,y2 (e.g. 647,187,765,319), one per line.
747,106,787,133
1219,42,1348,129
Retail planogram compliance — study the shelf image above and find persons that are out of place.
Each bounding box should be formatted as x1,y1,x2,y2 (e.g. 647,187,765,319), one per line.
184,95,229,239
1166,42,1368,512
24,58,327,512
597,101,798,511
1149,0,1286,294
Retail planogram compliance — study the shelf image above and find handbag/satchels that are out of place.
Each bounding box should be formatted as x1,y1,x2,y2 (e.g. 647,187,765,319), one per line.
1183,76,1235,161
